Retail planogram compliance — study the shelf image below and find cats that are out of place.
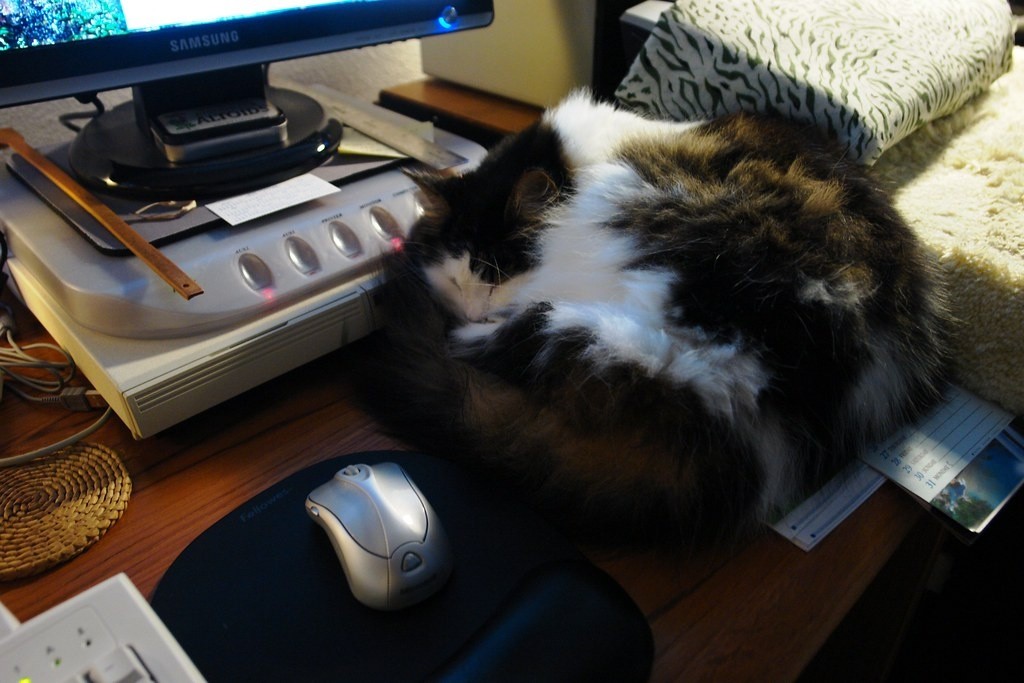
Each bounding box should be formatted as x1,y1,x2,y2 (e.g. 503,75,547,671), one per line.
348,85,962,556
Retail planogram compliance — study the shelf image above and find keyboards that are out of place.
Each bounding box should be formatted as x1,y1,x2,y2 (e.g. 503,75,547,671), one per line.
0,570,206,683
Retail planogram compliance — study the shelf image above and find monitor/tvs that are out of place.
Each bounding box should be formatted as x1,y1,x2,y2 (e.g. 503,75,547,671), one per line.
0,0,496,207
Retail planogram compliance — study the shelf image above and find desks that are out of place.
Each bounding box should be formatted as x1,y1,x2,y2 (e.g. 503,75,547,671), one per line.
381,46,1024,419
0,299,923,683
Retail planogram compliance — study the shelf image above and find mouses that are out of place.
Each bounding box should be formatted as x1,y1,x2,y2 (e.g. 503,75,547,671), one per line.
306,461,452,610
